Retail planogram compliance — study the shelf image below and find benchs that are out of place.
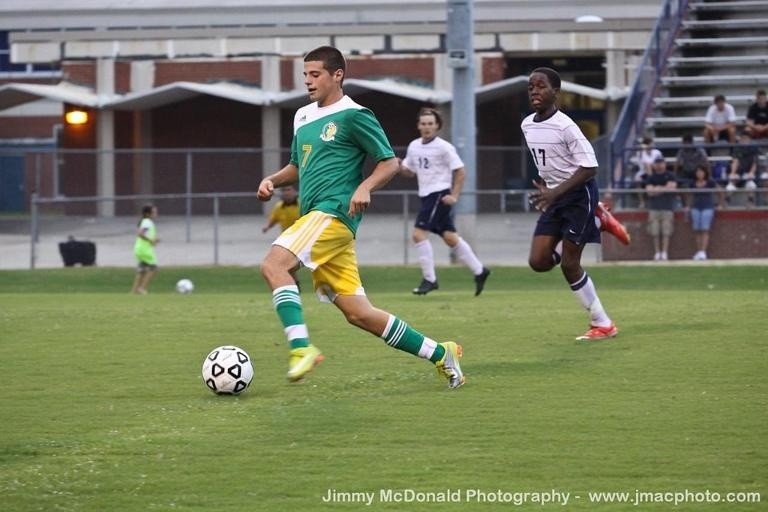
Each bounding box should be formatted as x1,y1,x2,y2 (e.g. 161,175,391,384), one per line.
627,0,767,203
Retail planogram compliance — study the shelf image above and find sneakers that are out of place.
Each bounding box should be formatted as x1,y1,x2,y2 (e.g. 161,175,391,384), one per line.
575,321,617,342
285,344,324,382
474,267,490,296
412,279,438,295
434,342,465,391
654,251,705,260
593,201,629,246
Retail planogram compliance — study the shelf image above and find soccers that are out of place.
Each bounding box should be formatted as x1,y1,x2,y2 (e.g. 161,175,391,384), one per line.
176,279,194,293
203,346,254,395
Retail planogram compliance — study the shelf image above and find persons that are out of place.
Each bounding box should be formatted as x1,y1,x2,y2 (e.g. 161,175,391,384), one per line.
397,107,491,296
255,45,466,390
520,67,631,342
130,203,161,294
622,89,768,261
262,182,301,296
